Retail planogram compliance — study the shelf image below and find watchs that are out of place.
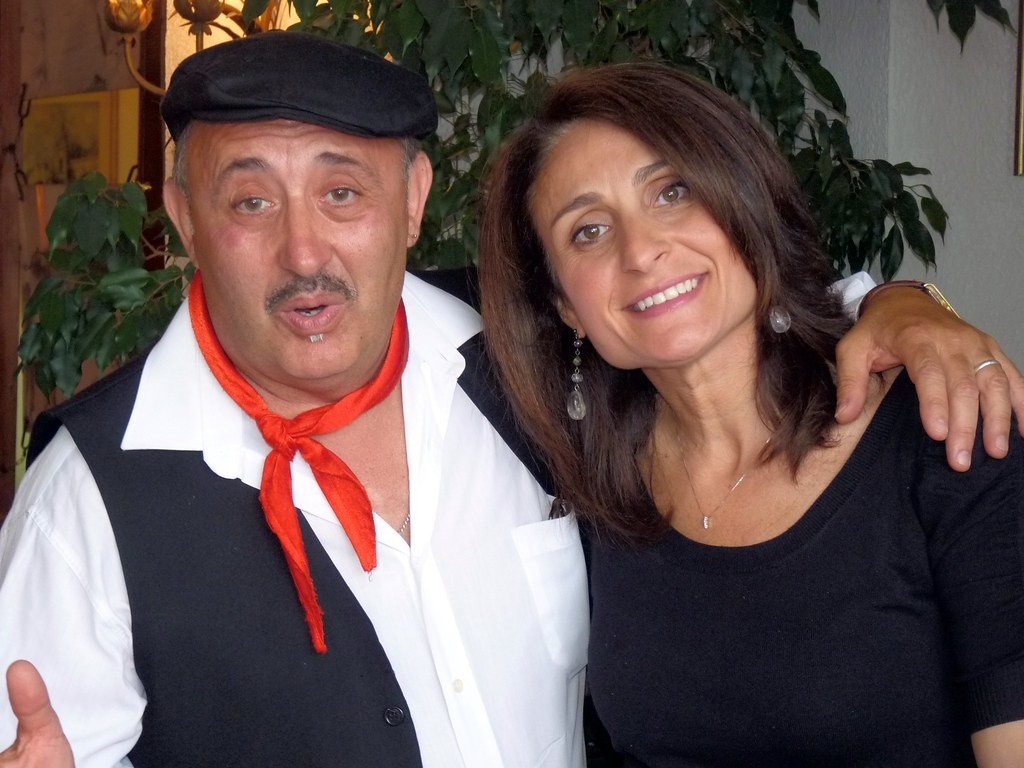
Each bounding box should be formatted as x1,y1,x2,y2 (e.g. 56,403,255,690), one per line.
855,273,966,322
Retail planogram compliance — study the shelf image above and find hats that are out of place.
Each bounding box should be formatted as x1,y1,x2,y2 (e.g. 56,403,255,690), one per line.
160,30,438,144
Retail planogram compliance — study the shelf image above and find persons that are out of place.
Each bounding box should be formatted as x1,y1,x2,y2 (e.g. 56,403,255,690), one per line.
0,29,1024,768
447,48,1023,768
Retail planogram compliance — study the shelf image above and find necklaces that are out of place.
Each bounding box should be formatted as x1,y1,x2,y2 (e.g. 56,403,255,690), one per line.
659,421,775,533
395,511,414,546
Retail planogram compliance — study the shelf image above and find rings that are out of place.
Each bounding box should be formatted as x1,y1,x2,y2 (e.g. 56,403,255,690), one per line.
973,352,1003,374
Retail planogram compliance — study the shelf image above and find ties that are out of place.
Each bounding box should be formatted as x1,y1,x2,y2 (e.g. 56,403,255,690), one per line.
188,268,409,654
